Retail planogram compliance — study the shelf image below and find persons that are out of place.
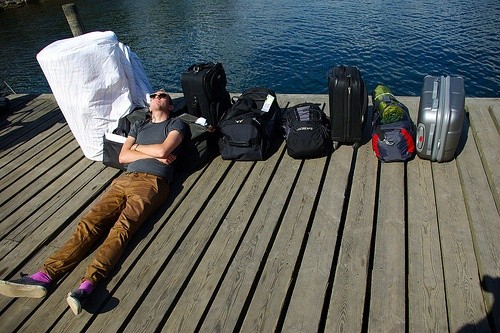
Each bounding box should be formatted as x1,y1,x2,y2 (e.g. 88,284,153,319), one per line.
0,89,185,315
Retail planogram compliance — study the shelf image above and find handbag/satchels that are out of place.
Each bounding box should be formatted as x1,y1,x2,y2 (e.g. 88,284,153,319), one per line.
370,84,416,163
169,97,187,116
281,101,333,159
217,88,283,161
103,107,215,177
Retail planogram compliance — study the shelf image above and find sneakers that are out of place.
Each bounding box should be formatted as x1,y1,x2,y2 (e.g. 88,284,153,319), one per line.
0,272,49,298
67,289,88,315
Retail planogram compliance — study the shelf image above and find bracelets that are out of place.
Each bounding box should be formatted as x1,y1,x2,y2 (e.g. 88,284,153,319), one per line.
134,144,139,151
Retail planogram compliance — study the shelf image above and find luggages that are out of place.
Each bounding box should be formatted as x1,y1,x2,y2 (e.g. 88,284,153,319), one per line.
328,64,368,149
181,61,231,135
416,75,465,162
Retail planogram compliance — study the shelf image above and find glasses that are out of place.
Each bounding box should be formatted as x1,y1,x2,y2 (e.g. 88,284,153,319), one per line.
150,93,167,98
